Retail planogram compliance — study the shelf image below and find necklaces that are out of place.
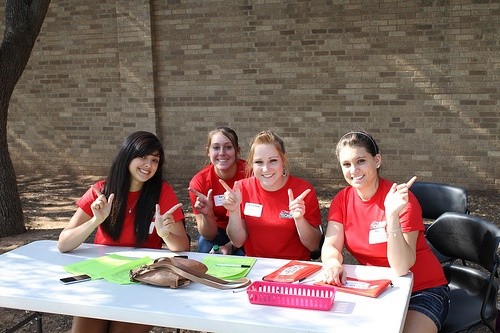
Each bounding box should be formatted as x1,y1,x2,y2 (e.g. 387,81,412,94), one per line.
126,197,138,214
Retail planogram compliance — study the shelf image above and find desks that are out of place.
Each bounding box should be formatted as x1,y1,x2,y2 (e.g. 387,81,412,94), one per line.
0,239,412,333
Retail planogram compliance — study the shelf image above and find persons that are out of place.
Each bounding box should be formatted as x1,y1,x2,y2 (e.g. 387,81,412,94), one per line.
188,126,255,257
57,131,191,333
217,130,321,261
321,131,452,333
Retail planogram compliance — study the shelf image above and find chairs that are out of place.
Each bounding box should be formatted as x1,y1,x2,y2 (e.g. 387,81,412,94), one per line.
410,180,470,231
425,212,500,332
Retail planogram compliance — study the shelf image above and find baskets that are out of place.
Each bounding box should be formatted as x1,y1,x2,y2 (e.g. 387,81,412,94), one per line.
247,281,336,311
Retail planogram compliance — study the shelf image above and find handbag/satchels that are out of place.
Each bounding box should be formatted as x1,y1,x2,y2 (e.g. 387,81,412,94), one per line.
129,256,209,288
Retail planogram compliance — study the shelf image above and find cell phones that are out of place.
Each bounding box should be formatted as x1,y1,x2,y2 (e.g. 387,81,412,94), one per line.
60,275,91,285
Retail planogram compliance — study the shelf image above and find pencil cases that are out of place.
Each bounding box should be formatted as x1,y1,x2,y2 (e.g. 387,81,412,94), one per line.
261,260,322,283
313,276,393,298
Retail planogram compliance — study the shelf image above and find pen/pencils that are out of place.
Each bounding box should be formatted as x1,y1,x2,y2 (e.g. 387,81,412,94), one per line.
300,280,315,283
291,277,306,284
216,264,250,267
